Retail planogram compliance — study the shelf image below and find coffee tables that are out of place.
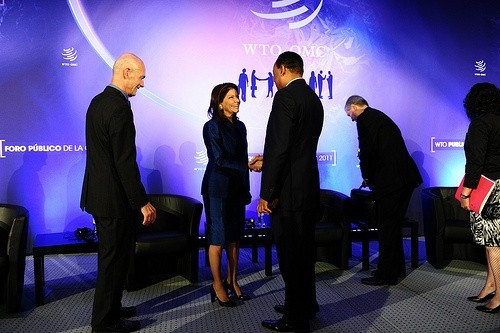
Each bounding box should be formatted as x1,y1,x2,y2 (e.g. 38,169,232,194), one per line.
32,230,99,300
351,218,419,272
203,219,273,275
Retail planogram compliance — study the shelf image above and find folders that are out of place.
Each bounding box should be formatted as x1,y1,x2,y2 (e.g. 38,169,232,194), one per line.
454,173,497,216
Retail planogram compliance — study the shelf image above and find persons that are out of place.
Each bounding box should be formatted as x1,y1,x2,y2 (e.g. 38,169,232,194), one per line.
343,94,424,286
252,50,327,333
457,82,500,314
80,54,157,332
198,82,265,306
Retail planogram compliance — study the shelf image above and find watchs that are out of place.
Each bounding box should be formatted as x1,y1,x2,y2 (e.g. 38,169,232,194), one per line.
460,193,469,199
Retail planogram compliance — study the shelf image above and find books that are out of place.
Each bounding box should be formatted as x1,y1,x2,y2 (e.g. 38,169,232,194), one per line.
455,173,495,214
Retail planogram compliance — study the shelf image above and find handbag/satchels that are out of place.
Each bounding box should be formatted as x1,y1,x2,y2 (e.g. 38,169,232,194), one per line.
351,184,378,224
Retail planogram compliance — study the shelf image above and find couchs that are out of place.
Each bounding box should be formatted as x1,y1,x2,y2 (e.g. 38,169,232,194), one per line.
420,187,485,270
125,193,203,291
317,188,353,270
0,204,29,308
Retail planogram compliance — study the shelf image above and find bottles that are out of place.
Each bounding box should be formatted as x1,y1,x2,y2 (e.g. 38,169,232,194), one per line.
92,218,97,239
258,212,265,225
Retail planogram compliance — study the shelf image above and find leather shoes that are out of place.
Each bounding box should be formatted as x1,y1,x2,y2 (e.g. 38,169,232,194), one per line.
274,304,316,320
121,307,137,317
372,271,405,278
92,319,141,333
261,318,311,333
361,276,399,286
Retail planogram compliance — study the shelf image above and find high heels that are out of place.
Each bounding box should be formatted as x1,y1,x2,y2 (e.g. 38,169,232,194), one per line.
468,291,496,303
475,305,500,313
209,284,237,306
223,279,250,300
